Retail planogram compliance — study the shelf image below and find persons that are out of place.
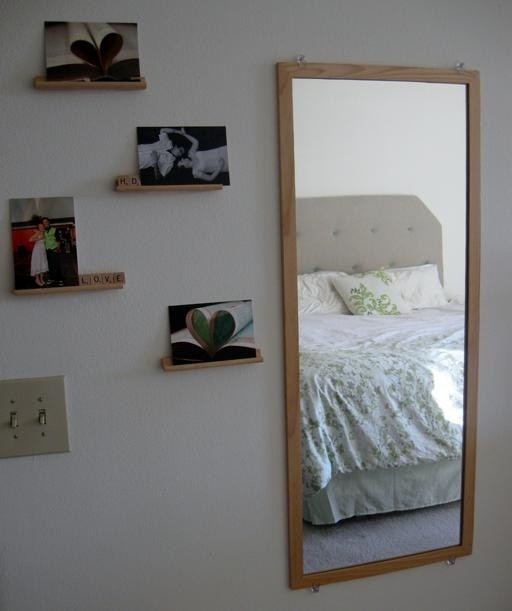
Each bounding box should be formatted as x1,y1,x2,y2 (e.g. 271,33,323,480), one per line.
28,223,49,286
33,217,65,286
138,128,185,181
175,128,229,181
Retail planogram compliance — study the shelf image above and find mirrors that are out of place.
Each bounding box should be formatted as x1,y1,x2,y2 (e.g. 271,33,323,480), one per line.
274,60,482,589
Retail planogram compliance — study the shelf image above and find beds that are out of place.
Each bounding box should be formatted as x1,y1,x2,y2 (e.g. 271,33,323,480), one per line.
295,194,465,526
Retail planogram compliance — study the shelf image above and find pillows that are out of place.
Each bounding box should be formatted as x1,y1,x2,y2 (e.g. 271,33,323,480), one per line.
297,263,453,317
